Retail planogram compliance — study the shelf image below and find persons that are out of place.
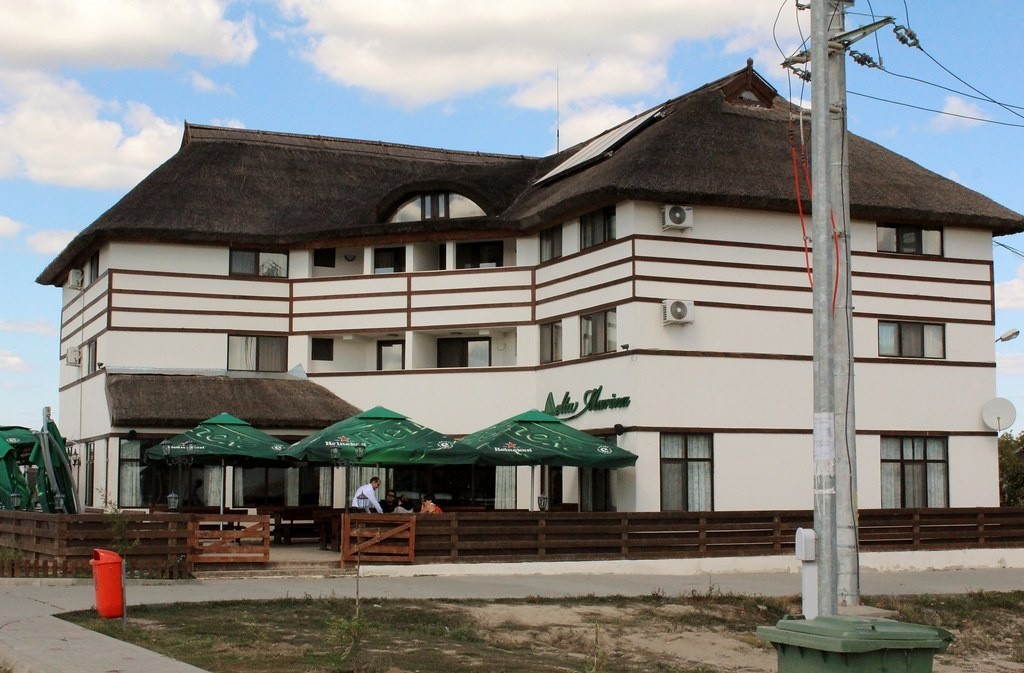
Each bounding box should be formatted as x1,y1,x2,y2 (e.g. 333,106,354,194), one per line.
378,490,399,513
417,492,443,513
186,477,206,509
349,474,384,515
392,494,414,514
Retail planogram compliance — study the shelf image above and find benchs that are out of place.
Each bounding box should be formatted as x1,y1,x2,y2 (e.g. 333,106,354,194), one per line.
274,506,331,543
181,508,248,546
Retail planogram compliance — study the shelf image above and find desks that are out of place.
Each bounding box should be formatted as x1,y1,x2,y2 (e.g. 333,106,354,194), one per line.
313,509,345,553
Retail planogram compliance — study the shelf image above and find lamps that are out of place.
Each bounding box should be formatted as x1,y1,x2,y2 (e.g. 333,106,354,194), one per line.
67,448,71,462
167,490,180,513
72,448,78,465
537,493,548,509
357,491,368,511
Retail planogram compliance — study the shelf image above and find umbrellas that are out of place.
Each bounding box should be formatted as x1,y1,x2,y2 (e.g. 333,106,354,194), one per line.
141,411,294,542
276,405,488,518
0,408,80,517
459,408,637,509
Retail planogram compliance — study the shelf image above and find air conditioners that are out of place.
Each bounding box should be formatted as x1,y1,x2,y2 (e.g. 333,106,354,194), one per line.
661,204,693,231
663,299,695,324
66,346,78,364
67,270,81,289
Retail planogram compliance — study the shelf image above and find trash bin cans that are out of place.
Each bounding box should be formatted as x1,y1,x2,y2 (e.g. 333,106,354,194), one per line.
89,549,124,619
757,614,954,673
